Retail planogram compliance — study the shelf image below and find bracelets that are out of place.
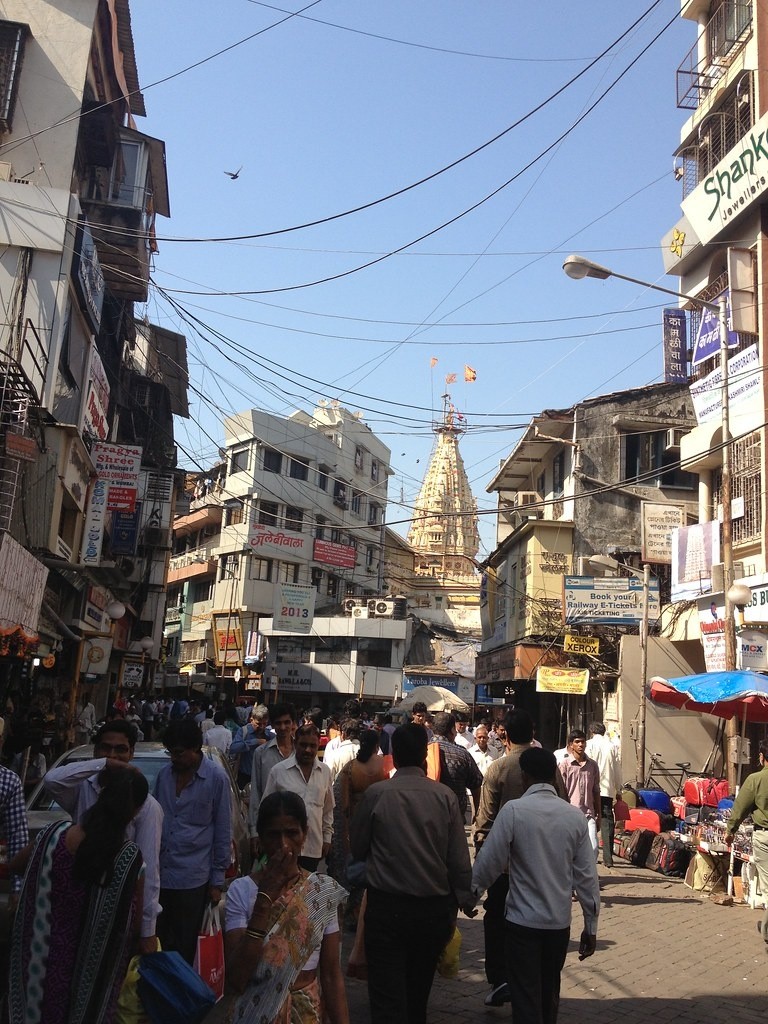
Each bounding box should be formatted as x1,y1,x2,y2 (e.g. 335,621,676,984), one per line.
256,892,272,903
246,933,264,941
246,929,265,937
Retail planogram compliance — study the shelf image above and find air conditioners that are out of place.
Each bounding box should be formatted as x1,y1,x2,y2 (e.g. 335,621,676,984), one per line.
699,57,732,100
712,562,744,593
345,599,363,612
367,599,384,612
142,527,170,551
316,571,323,578
120,557,141,582
375,601,395,616
666,429,691,452
352,607,370,619
514,491,544,516
227,556,238,563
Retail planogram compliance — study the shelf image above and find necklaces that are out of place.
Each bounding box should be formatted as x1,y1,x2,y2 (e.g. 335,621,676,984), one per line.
288,867,303,888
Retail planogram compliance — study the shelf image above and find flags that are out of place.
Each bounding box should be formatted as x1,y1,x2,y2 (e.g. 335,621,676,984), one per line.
431,358,438,368
446,373,457,384
466,365,476,381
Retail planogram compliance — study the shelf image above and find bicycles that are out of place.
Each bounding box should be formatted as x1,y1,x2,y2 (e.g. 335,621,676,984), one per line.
620,753,711,797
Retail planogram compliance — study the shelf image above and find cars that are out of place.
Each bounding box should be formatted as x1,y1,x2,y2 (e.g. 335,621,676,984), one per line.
18,742,254,925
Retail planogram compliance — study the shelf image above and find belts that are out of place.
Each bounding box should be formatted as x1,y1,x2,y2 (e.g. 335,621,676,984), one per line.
755,827,768,830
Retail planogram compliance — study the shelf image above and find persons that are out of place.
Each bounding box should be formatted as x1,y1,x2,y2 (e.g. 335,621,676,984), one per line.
470,709,601,1024
723,739,768,944
707,601,723,623
585,722,623,868
0,689,350,1023
323,696,474,1024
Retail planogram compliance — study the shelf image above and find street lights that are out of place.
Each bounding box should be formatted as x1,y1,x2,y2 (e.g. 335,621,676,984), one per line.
360,666,368,699
588,555,651,808
194,556,235,694
562,254,737,798
115,636,154,704
66,601,125,732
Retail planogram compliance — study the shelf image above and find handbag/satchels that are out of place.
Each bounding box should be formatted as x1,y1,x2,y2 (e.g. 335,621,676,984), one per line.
437,925,462,979
114,937,218,1024
614,775,730,876
192,902,225,1003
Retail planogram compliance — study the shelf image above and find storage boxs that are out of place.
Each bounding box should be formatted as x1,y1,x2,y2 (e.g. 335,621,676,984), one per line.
733,877,743,899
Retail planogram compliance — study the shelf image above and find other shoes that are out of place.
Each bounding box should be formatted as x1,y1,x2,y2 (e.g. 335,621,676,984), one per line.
347,960,367,980
485,982,509,1007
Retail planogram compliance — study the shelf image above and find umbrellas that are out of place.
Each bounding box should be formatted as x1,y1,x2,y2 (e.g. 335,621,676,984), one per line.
396,685,470,712
649,666,768,911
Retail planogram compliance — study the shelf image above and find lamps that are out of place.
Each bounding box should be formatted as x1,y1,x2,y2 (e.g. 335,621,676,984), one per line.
674,146,720,181
270,662,277,671
699,112,746,149
736,69,768,110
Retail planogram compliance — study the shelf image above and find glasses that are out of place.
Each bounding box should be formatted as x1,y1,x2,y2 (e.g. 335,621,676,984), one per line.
96,742,131,755
163,748,193,757
